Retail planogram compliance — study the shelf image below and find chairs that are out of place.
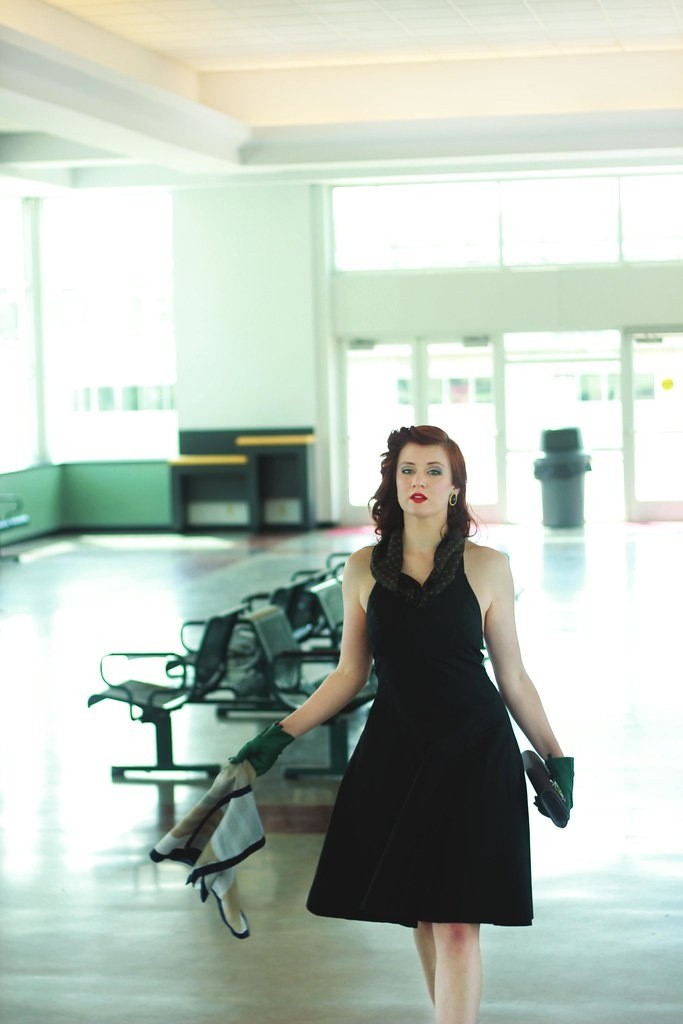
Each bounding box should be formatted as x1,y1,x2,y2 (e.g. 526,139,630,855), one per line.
86,552,379,780
0,494,33,564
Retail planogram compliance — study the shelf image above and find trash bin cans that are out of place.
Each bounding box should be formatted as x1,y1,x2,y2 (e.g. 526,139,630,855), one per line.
534,429,591,529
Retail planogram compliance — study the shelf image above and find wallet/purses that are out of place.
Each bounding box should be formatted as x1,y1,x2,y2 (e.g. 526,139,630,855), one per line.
522,750,568,828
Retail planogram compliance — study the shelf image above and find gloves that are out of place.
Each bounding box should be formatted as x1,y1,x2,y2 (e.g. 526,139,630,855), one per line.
531,755,574,820
227,719,294,778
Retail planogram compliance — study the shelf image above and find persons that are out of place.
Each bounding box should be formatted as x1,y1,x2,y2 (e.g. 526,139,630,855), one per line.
227,426,575,1023
